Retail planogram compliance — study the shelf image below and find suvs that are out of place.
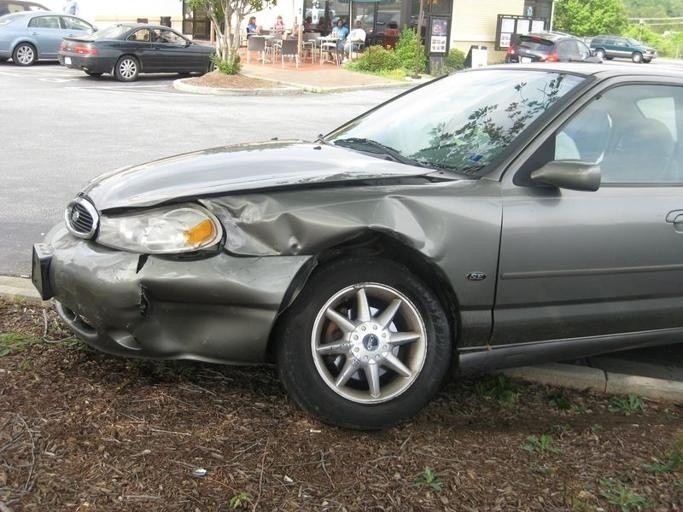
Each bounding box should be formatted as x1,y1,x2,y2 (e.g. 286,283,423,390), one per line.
502,29,658,67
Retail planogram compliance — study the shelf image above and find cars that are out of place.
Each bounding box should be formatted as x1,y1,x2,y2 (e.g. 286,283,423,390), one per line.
56,22,223,82
26,60,683,438
0,0,99,67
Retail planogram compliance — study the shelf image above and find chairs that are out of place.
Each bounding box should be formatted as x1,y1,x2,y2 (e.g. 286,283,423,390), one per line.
570,110,680,183
127,33,160,43
245,21,368,68
32,18,70,28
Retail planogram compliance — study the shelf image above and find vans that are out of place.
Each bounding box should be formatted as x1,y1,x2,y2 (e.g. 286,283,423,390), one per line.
306,8,402,32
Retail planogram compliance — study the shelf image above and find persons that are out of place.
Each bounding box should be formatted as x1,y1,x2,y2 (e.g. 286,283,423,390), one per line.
273,15,286,35
247,16,263,37
340,19,368,64
325,18,348,64
291,15,333,48
